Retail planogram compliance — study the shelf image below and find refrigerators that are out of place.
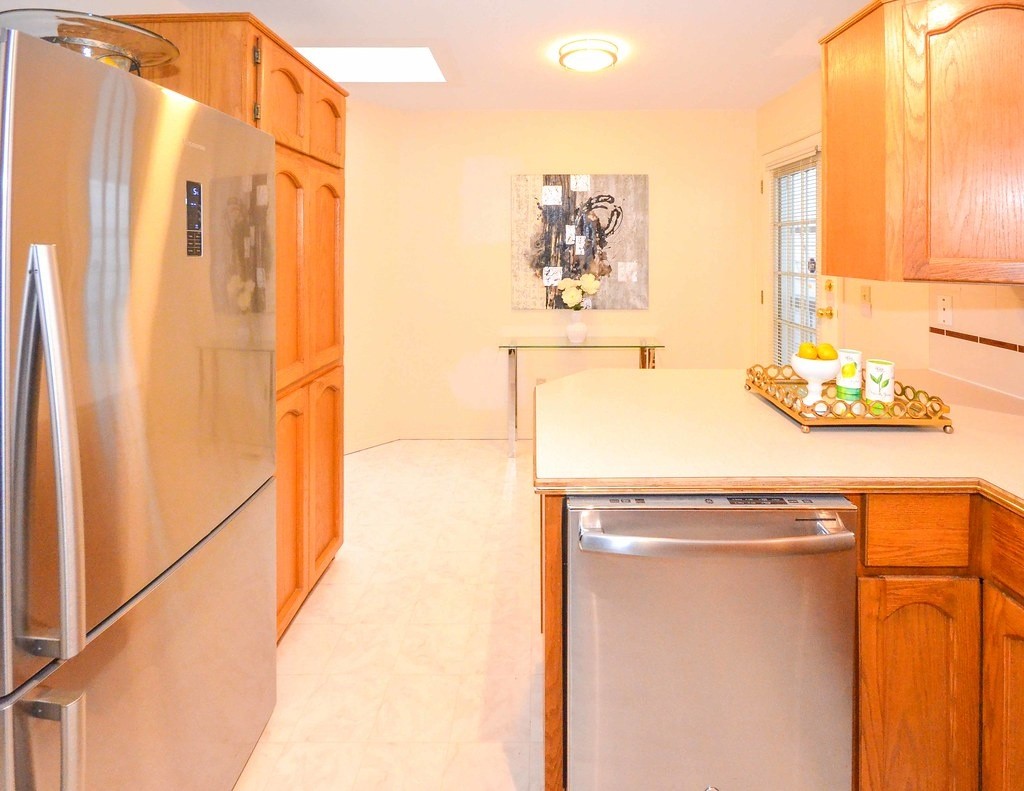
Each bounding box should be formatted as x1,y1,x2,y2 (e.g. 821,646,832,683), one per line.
0,29,277,791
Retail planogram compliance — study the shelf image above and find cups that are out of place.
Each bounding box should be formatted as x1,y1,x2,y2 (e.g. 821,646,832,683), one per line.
837,348,862,415
865,359,896,418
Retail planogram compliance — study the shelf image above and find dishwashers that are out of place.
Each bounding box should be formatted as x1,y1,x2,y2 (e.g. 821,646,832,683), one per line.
563,493,860,791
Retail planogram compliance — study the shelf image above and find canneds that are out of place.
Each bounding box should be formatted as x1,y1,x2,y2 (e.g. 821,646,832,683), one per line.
836,348,862,415
864,359,895,416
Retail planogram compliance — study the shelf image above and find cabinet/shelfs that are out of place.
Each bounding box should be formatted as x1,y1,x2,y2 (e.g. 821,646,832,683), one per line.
59,10,344,645
854,481,981,791
817,0,1023,284
979,484,1023,791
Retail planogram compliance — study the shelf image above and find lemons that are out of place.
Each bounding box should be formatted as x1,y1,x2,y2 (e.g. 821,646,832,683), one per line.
841,363,856,378
796,341,838,360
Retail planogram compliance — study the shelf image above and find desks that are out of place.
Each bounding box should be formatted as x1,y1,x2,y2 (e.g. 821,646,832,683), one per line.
498,339,666,456
